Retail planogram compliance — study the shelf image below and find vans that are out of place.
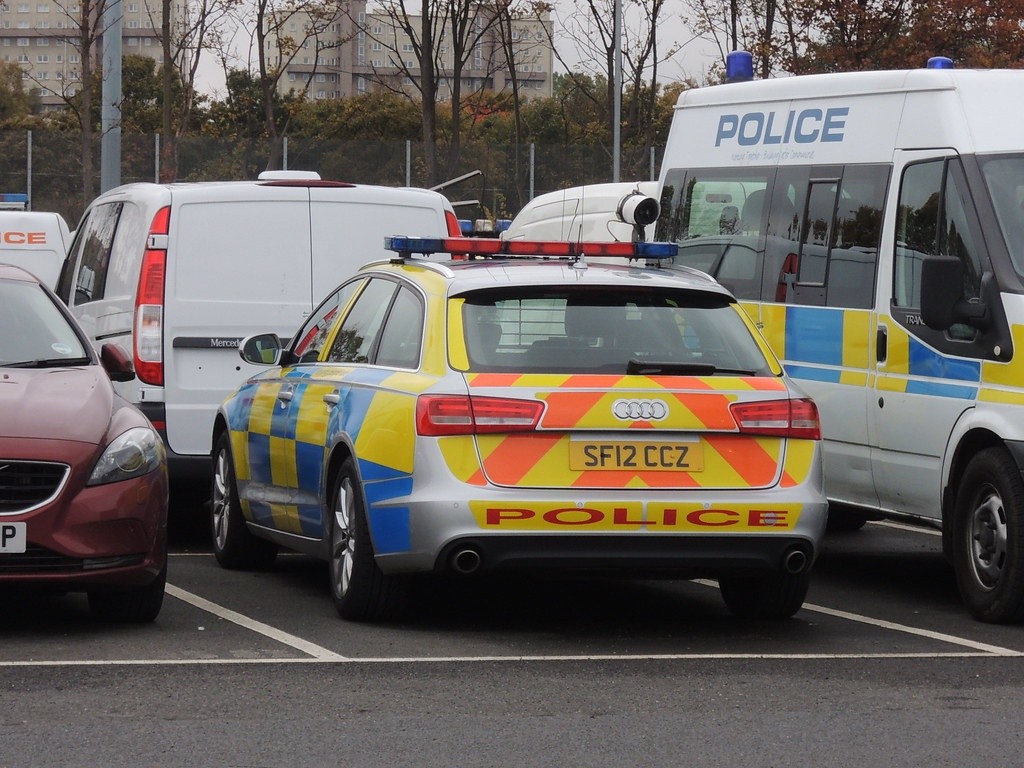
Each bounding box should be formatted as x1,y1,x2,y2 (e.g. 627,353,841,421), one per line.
55,168,472,512
493,50,1024,625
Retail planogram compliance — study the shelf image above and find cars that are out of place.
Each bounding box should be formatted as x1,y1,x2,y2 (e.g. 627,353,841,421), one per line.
212,235,829,650
1,194,171,625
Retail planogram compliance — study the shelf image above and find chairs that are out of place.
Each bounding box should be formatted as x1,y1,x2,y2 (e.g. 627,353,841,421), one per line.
480,322,502,363
564,299,630,357
740,189,794,233
